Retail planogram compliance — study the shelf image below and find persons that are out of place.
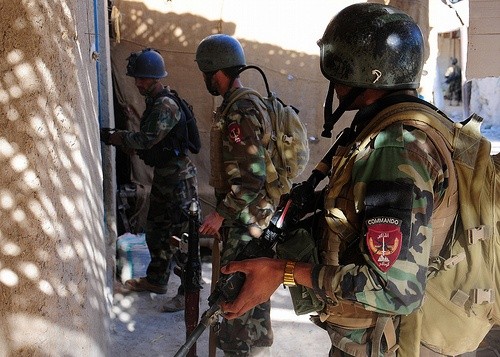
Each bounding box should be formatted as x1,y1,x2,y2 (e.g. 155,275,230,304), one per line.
221,3,462,357
193,34,276,357
444,57,462,106
101,50,202,310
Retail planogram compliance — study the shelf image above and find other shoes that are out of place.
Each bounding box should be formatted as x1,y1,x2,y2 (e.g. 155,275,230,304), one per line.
158,293,185,313
124,276,169,295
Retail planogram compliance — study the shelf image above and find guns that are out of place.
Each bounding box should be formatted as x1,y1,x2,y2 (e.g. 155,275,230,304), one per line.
175,127,359,357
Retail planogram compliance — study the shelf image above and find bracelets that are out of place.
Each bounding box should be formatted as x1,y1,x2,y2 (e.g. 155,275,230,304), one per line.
283,261,296,287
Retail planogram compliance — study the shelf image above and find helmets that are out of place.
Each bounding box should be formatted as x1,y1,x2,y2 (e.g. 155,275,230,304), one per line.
193,34,247,73
316,2,425,90
451,58,457,65
125,48,169,79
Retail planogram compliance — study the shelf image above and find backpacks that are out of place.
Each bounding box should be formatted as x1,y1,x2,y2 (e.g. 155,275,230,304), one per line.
146,90,202,154
322,100,500,357
209,87,311,179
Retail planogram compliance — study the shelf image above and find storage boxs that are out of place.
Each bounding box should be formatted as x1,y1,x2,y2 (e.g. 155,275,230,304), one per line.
116,233,151,285
